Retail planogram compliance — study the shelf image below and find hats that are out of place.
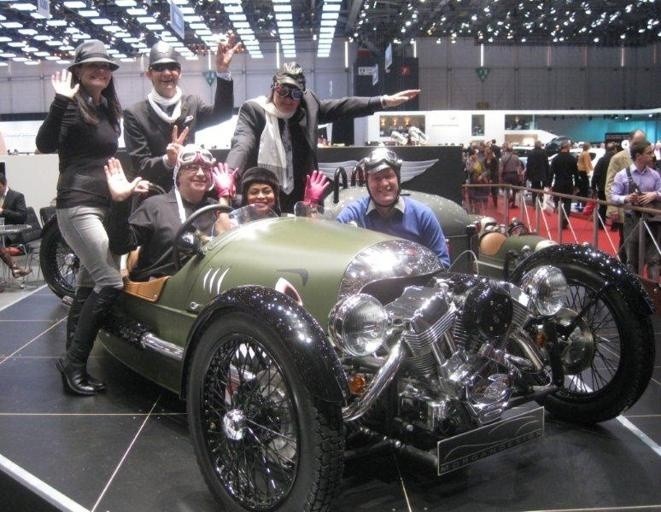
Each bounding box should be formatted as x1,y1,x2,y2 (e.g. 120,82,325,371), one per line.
149,41,180,65
364,148,401,175
273,61,307,92
177,143,216,167
242,167,280,190
65,39,120,73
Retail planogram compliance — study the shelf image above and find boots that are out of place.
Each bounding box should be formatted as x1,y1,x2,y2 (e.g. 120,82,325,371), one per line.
55,285,123,396
67,287,108,393
2,252,33,279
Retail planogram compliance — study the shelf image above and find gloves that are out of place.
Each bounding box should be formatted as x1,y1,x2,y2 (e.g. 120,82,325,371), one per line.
211,161,240,199
303,170,330,207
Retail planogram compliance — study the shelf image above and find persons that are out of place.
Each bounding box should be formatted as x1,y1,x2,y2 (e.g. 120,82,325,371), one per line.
0,241,32,278
0,174,28,245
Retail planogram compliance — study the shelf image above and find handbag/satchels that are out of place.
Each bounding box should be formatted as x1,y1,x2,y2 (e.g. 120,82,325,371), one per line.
541,193,557,215
581,194,598,217
627,182,659,220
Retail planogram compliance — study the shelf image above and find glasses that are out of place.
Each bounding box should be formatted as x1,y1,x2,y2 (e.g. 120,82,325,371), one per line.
180,149,214,166
276,87,302,100
148,61,180,73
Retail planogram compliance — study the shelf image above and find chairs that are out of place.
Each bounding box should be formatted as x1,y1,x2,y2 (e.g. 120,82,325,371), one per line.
1,204,56,289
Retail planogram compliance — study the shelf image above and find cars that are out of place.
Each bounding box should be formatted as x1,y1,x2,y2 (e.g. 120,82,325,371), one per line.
545,146,608,185
309,165,561,284
37,164,657,512
498,146,535,166
